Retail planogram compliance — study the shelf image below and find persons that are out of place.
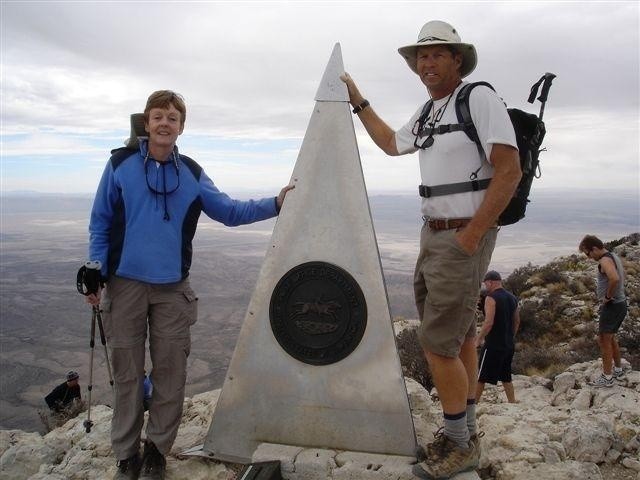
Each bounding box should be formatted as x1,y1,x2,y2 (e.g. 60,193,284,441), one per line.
143,366,152,411
473,270,520,404
579,235,628,388
84,90,295,480
339,20,522,479
45,371,82,418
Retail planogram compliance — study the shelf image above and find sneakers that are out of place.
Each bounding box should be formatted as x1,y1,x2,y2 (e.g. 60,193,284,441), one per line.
587,373,613,388
141,439,166,480
412,429,479,479
611,370,625,381
112,447,139,480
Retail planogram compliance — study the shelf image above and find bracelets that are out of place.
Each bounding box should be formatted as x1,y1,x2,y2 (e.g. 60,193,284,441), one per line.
353,100,368,114
605,296,611,300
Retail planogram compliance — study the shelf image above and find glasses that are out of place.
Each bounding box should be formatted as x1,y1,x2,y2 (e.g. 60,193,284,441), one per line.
586,250,594,256
413,128,434,149
145,172,180,194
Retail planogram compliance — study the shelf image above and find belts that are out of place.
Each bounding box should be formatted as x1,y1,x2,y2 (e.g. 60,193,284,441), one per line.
420,216,474,231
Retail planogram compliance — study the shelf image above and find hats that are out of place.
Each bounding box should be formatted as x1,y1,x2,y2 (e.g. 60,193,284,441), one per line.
396,16,479,77
65,370,80,381
127,113,145,149
482,269,501,282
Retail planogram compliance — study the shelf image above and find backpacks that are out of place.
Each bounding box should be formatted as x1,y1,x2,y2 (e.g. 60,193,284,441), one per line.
497,108,546,225
